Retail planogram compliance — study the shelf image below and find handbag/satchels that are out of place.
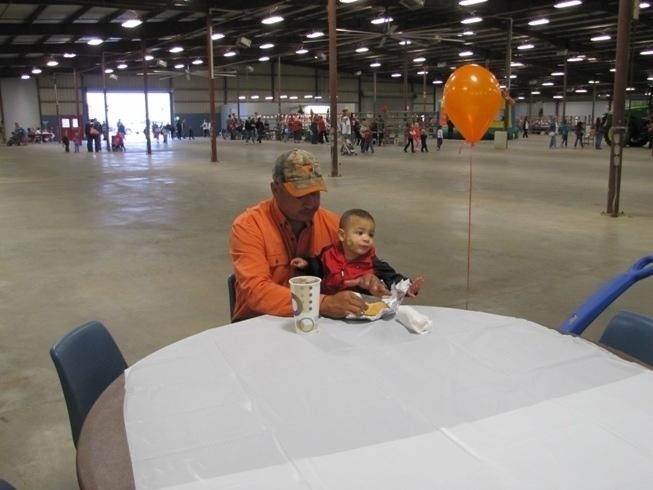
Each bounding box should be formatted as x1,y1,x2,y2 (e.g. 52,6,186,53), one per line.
90,126,100,135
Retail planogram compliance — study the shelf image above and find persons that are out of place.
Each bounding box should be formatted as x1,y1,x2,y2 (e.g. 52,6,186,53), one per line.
289,208,424,300
62,119,106,153
522,116,529,138
222,113,264,144
152,120,195,144
548,117,607,150
200,119,210,138
14,122,40,145
340,110,385,153
116,119,126,152
403,112,453,152
279,113,329,144
228,148,369,325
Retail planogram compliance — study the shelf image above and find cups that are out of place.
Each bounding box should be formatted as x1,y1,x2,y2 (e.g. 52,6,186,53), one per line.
288,275,322,334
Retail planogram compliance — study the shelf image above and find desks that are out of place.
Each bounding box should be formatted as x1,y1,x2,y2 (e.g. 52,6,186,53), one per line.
77,305,653,489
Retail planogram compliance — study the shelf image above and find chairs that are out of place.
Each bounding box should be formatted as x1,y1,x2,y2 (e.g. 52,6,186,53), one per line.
599,309,653,367
49,320,127,450
227,274,237,313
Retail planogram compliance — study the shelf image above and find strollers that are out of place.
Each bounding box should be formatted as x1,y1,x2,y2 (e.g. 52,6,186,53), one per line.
340,136,357,157
110,133,124,153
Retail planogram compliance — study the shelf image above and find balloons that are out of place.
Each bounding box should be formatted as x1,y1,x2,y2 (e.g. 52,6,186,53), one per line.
441,63,502,149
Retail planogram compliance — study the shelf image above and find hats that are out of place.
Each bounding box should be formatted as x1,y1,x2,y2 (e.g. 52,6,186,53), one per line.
272,148,329,198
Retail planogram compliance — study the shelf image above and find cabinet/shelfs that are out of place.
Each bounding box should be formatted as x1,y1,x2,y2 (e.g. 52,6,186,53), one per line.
261,111,411,146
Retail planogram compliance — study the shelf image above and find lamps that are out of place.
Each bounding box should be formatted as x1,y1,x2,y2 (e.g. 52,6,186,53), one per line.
19,11,206,82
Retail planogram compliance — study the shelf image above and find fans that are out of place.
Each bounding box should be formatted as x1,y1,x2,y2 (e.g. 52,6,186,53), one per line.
137,66,237,80
302,7,466,49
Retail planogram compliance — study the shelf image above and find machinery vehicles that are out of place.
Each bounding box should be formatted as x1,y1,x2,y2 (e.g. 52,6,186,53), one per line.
601,90,652,149
